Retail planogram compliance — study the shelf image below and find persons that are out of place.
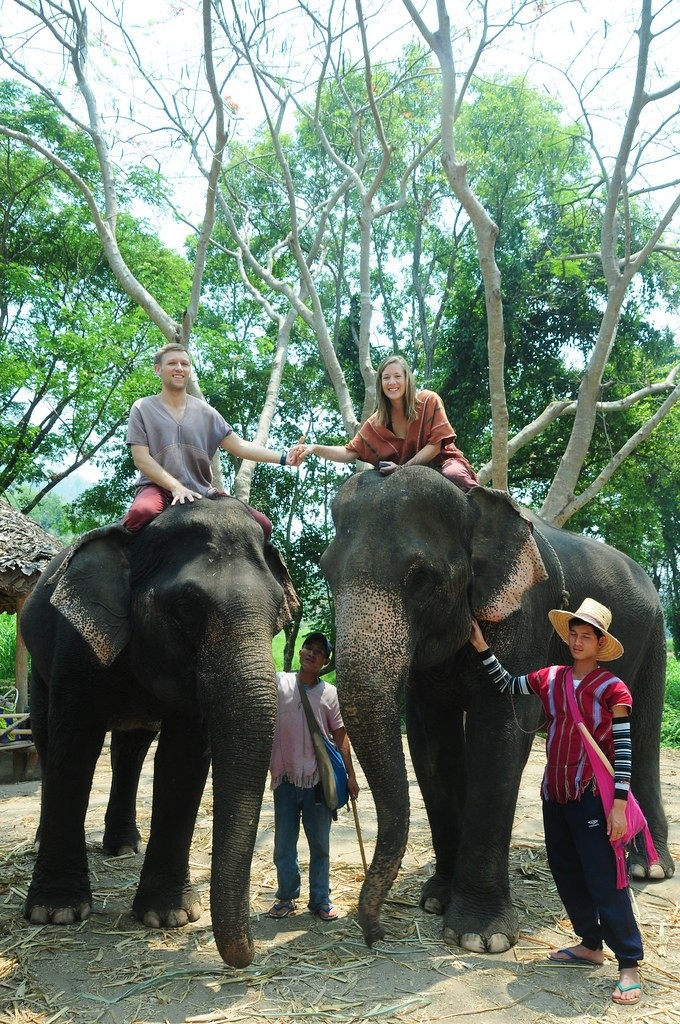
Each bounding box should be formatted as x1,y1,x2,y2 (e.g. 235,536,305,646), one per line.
288,355,480,493
120,343,304,535
269,632,360,920
471,597,645,1005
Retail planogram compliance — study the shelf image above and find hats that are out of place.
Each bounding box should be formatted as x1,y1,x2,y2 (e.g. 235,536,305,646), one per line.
547,598,624,663
302,633,333,659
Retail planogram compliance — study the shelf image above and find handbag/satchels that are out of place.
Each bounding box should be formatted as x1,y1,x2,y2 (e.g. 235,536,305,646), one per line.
311,731,349,811
578,722,660,889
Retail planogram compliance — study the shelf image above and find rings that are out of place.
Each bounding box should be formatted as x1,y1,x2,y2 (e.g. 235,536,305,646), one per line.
617,832,622,834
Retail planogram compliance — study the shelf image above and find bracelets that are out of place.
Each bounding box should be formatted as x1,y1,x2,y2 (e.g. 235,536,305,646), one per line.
401,465,404,470
280,450,287,467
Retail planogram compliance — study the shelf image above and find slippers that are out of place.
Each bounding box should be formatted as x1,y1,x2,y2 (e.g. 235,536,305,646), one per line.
612,979,641,1005
317,904,339,920
547,947,599,965
269,901,296,918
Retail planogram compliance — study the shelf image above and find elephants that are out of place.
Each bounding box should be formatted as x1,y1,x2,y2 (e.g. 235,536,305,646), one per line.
318,463,677,957
18,493,300,968
